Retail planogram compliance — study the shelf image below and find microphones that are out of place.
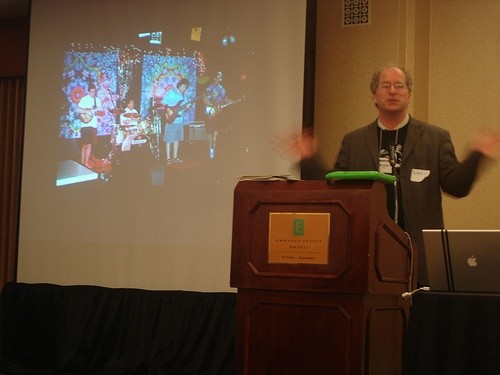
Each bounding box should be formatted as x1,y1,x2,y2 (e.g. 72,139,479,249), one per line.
386,140,396,166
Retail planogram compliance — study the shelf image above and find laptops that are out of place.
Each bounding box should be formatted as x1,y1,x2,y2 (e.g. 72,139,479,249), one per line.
422,229,500,293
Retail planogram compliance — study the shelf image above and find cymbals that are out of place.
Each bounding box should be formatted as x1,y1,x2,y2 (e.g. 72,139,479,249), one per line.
109,108,125,114
124,113,140,119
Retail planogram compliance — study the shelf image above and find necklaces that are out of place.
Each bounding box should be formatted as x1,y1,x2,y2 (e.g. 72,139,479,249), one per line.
379,123,399,157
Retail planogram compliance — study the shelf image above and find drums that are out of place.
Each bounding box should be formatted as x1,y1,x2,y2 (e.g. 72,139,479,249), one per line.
119,134,151,153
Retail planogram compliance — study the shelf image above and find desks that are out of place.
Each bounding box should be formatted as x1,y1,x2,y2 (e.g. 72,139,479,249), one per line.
407,290,500,375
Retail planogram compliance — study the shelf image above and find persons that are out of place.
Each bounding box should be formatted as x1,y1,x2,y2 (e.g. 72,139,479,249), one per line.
203,73,233,152
270,64,492,290
120,99,139,124
77,84,102,168
161,78,192,163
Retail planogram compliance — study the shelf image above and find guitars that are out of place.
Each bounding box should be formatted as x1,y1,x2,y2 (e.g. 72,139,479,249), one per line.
77,96,118,123
205,95,245,119
162,92,203,123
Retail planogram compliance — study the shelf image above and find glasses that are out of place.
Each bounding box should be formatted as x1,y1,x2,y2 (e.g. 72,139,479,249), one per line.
377,82,405,91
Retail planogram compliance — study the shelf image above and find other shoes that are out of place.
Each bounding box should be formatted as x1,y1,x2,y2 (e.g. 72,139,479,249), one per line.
167,158,183,165
86,164,92,169
